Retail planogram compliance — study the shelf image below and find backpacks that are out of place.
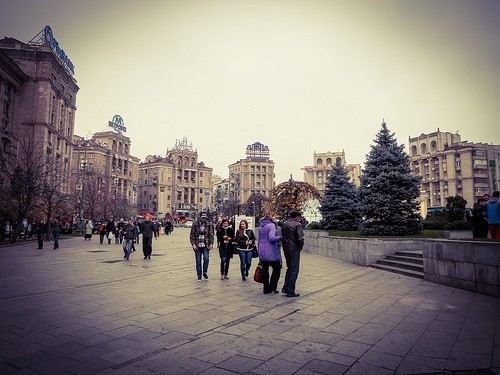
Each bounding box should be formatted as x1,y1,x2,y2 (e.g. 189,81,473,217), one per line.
465,205,483,223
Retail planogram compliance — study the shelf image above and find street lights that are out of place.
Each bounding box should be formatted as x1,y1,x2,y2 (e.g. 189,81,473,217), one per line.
252,201,255,216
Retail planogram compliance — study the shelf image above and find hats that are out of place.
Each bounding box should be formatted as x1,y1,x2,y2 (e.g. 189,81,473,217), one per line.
200,212,209,219
290,210,301,218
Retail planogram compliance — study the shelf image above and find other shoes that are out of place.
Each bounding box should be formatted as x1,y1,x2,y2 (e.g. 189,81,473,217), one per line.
263,289,272,294
197,277,201,282
144,257,147,259
282,285,287,293
224,276,229,279
203,273,208,279
243,276,246,281
221,275,224,280
126,257,129,260
269,286,279,293
148,256,151,259
124,255,126,258
245,268,248,277
287,291,300,297
279,264,282,268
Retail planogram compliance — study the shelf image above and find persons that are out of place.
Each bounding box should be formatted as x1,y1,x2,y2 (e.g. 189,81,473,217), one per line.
281,212,304,296
84,217,174,259
19,218,63,249
215,218,235,279
470,191,500,239
258,212,282,294
235,220,256,281
190,212,214,281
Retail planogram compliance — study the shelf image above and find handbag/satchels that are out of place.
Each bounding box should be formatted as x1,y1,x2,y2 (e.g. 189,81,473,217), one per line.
252,240,259,258
254,259,264,283
108,232,113,240
